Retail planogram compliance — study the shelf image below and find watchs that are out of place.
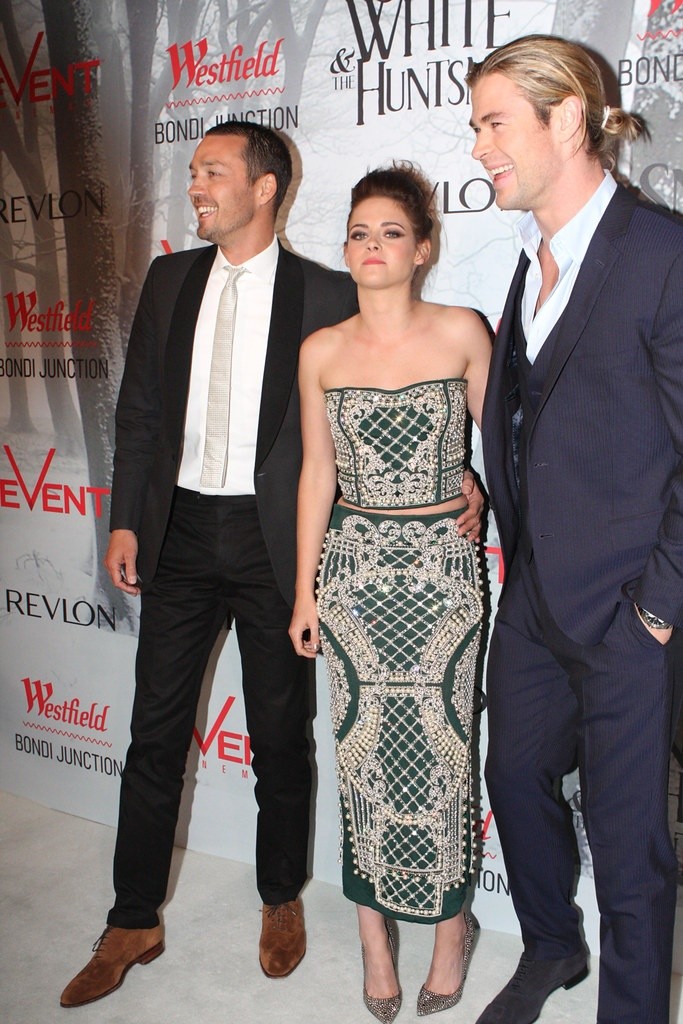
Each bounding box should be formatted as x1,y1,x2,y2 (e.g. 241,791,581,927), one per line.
636,602,670,629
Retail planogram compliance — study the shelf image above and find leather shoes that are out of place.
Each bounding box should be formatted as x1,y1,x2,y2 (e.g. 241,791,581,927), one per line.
59,925,165,1008
258,897,307,979
474,944,589,1024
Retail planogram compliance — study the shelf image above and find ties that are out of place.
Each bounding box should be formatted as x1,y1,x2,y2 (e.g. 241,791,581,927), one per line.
197,264,243,489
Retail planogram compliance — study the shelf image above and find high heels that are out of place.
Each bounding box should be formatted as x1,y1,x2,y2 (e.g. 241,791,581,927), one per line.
415,911,477,1017
361,917,403,1024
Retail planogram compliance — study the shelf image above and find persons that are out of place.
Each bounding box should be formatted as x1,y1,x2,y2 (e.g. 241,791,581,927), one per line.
58,122,484,1009
288,158,492,1024
466,37,683,1024
